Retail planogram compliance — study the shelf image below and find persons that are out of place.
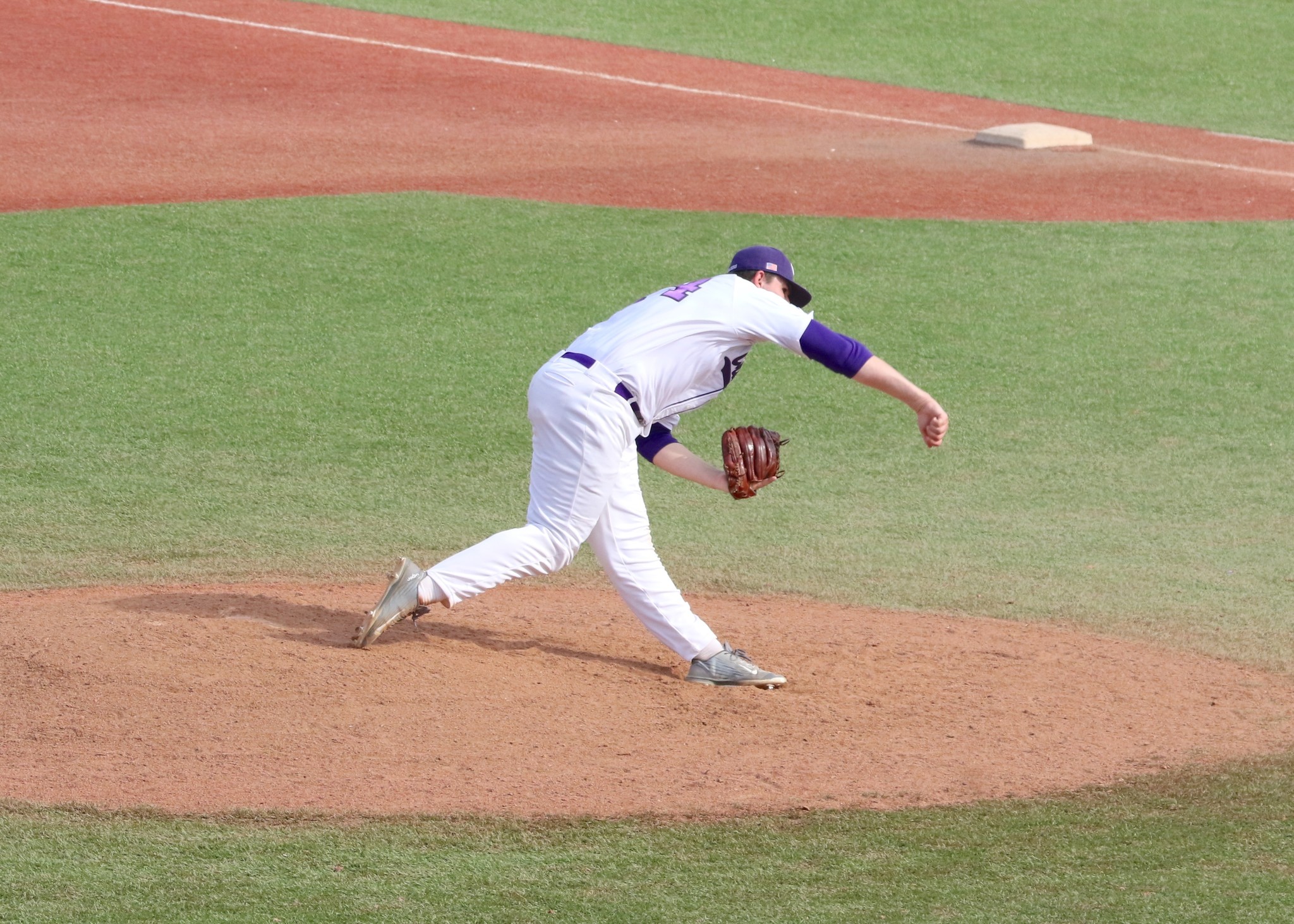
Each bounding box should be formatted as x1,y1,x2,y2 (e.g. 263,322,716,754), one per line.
348,246,948,687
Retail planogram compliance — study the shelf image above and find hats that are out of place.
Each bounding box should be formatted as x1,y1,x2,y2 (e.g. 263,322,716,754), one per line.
728,246,812,308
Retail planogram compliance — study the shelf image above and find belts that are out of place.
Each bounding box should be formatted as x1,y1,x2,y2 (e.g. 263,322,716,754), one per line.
559,351,643,420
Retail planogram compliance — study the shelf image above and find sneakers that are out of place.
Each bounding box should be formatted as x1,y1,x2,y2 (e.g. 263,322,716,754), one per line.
684,641,787,688
354,557,431,648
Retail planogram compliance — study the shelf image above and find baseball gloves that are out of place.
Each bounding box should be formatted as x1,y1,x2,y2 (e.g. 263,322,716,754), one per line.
721,425,781,500
